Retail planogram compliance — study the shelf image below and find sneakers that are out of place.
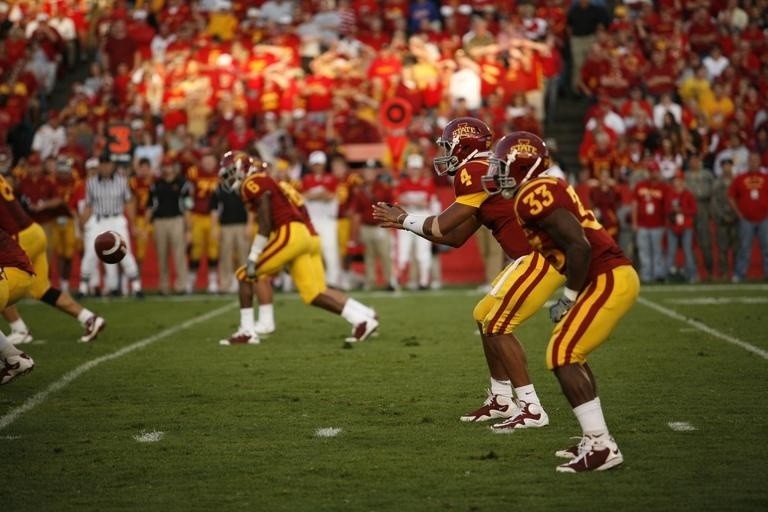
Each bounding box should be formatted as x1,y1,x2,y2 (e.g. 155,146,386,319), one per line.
344,309,380,343
0,352,35,385
218,320,275,346
554,433,623,472
459,393,550,433
80,314,106,342
8,329,33,344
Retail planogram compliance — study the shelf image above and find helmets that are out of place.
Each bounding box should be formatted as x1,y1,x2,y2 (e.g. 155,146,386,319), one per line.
218,149,267,193
480,130,549,201
432,117,493,177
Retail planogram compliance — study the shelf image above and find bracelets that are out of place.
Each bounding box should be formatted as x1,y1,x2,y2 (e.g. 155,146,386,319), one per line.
402,214,429,237
247,234,268,263
396,213,406,224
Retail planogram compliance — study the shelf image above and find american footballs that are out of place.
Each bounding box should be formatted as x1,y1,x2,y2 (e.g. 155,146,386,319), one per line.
94,232,127,263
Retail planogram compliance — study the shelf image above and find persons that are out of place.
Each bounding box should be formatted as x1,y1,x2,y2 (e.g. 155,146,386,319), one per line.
1,231,39,386
217,150,379,349
480,132,640,477
2,2,767,304
0,172,107,348
230,153,376,338
372,115,568,433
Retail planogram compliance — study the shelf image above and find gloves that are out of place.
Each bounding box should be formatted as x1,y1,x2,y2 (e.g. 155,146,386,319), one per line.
548,294,575,323
244,259,257,280
